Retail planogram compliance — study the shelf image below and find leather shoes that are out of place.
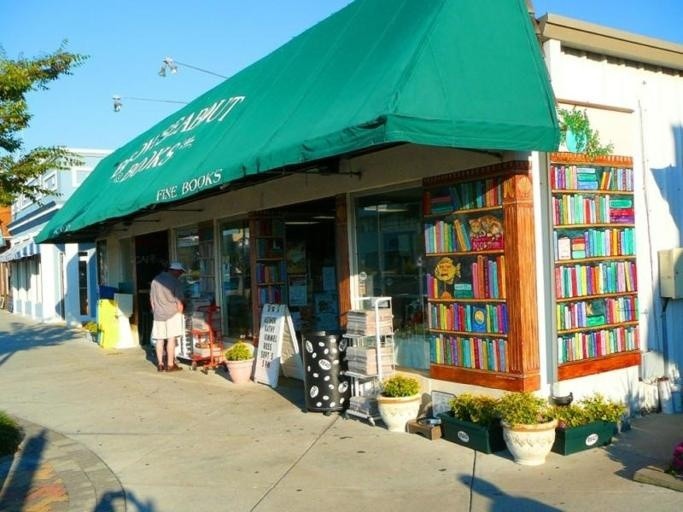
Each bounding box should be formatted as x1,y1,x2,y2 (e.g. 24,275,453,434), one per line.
158,364,183,372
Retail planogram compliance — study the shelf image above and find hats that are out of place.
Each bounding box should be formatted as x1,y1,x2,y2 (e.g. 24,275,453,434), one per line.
169,262,186,272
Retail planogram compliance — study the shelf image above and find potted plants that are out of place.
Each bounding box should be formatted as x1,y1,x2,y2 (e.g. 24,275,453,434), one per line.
556,105,614,158
437,392,508,453
553,393,626,456
497,391,559,465
224,342,256,384
376,373,422,431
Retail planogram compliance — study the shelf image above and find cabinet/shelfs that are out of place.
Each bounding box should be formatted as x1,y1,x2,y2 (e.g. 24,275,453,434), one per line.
422,161,540,393
192,303,226,374
359,199,429,299
550,151,640,380
344,296,395,427
251,214,289,347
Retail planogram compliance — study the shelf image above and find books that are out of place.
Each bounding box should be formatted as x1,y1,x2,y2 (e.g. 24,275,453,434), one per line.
552,193,635,225
427,302,508,334
558,325,639,364
255,220,285,306
197,243,215,296
556,261,636,298
426,217,471,253
557,294,638,330
554,227,636,262
426,333,510,372
428,255,506,299
550,164,634,191
426,177,503,215
345,308,394,416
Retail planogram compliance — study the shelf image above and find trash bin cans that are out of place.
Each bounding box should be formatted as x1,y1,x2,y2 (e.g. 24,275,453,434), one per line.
301,329,350,416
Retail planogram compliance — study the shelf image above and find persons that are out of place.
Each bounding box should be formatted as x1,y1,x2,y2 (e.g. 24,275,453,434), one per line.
149,262,187,372
